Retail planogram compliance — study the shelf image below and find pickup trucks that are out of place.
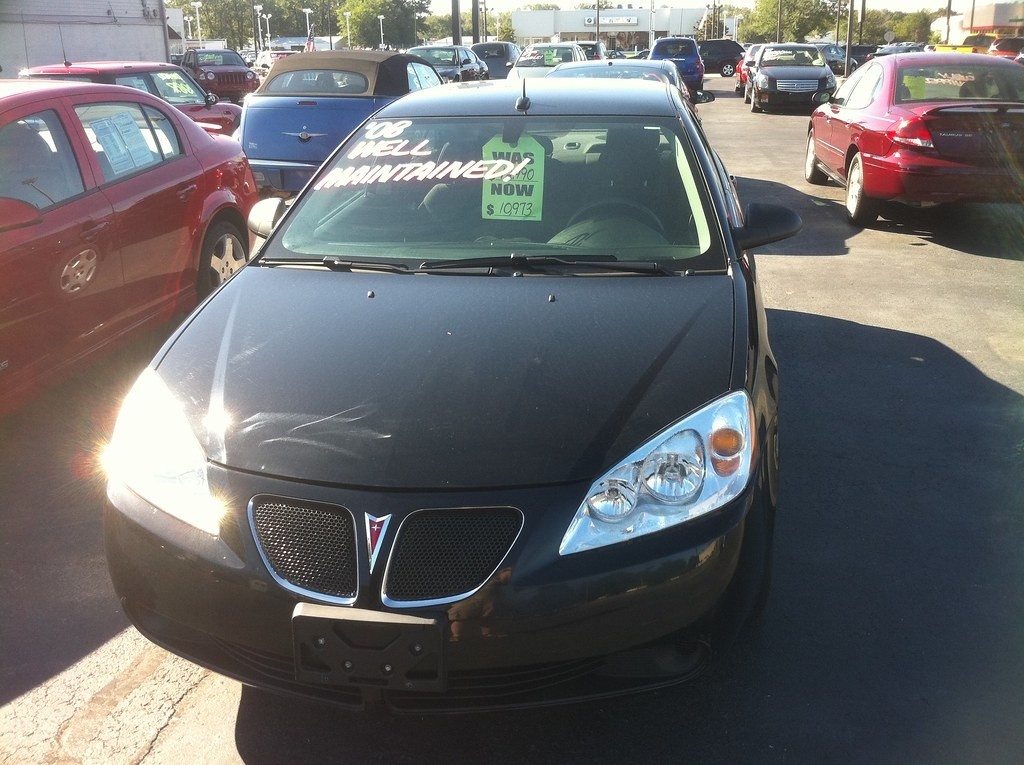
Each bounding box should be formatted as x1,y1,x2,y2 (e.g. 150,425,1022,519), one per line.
932,33,1014,54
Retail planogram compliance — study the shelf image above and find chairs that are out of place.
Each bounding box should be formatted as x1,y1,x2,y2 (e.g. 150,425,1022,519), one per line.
562,53,571,61
681,46,691,55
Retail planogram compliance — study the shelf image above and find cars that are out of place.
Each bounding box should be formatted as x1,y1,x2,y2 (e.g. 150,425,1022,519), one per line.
696,39,746,77
989,37,1024,58
471,41,715,114
102,78,805,717
735,43,837,113
0,79,260,419
815,42,935,75
238,50,299,69
407,46,489,84
238,50,447,192
17,60,243,136
804,52,1024,228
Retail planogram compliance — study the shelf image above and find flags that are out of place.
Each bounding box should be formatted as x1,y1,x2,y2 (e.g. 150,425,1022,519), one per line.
303,27,315,52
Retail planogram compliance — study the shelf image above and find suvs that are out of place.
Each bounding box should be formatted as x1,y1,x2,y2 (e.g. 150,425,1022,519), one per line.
180,47,261,104
647,36,704,90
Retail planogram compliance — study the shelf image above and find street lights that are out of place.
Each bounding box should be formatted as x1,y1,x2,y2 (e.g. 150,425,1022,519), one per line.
480,8,488,42
722,10,743,41
592,5,607,41
184,1,386,51
706,4,723,39
